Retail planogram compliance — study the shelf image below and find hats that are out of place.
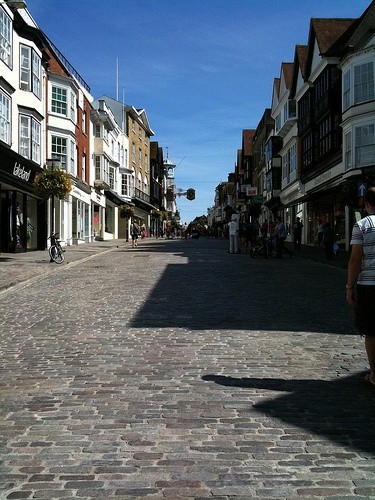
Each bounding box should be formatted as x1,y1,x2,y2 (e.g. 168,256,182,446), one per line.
232,218,236,220
134,220,137,222
297,217,301,219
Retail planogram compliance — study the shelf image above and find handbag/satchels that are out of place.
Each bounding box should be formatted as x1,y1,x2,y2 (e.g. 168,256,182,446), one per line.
133,228,137,233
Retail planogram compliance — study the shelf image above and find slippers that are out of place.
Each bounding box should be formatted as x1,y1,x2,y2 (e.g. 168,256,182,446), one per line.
363,374,375,386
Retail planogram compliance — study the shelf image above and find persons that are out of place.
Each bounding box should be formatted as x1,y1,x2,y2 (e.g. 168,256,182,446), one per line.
130,219,146,248
16,206,32,249
294,217,304,252
228,217,294,259
166,222,221,240
314,219,333,262
345,188,375,384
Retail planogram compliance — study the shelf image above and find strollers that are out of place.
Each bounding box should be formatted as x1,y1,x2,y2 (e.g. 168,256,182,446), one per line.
249,232,278,258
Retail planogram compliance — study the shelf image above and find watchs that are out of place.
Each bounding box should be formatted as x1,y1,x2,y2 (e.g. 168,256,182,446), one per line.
346,284,354,289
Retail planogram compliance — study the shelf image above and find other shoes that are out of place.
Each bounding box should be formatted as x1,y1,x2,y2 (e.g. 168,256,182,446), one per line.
289,252,294,258
274,256,282,259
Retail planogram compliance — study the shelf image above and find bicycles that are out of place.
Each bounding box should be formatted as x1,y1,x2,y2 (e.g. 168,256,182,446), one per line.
46,230,66,264
